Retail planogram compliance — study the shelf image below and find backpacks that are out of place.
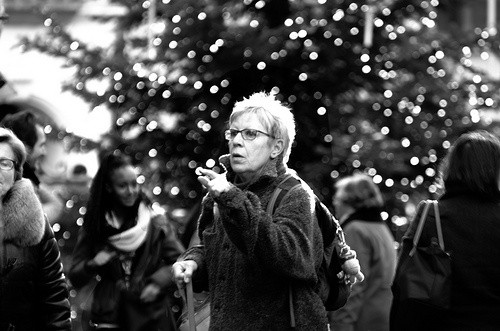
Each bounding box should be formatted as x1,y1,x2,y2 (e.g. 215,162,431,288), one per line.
213,176,365,311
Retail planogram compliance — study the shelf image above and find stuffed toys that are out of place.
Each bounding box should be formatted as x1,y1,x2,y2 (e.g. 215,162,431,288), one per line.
337,245,364,284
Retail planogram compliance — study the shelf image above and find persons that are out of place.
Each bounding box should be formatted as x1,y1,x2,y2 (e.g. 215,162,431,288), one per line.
170,91,338,331
67,152,185,331
0,110,46,188
389,130,500,331
331,175,397,331
0,127,71,331
61,165,90,225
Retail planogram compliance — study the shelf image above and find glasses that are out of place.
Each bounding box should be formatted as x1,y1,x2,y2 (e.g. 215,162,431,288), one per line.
225,129,274,141
0,158,17,170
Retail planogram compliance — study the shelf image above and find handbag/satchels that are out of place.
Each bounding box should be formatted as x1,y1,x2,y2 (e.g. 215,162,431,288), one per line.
391,199,453,318
